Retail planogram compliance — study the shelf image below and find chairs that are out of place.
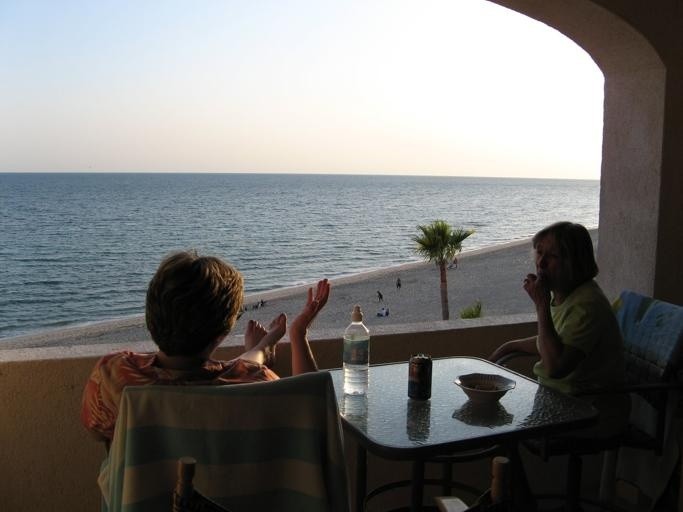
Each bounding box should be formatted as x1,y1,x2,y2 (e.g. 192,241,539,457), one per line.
499,289,683,512
96,369,352,512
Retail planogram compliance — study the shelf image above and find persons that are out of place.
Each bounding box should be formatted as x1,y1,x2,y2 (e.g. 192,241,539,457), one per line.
453,257,457,267
488,221,626,443
79,249,331,460
377,290,384,301
396,277,402,291
376,306,390,318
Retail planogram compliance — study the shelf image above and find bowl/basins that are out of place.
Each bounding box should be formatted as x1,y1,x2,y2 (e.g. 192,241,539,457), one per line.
453,372,517,402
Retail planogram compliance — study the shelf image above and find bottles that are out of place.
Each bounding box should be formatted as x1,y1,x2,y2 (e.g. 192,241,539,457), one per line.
342,306,371,396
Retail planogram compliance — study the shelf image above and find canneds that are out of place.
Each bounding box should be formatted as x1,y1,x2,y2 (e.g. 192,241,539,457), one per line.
408,351,431,400
